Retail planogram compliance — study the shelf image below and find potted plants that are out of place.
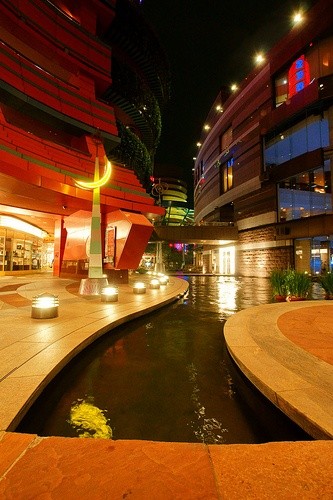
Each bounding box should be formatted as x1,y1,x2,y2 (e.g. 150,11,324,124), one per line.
317,272,333,300
286,273,310,301
268,267,289,302
